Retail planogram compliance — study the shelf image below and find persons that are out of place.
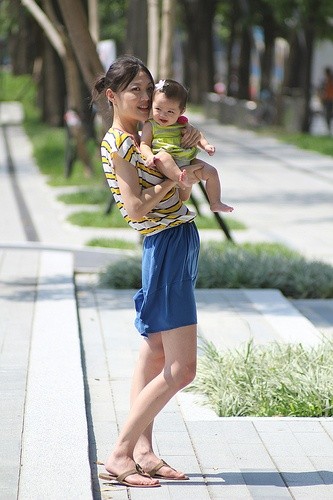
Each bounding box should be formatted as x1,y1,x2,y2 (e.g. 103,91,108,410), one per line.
61,108,96,179
99,57,234,488
318,67,333,128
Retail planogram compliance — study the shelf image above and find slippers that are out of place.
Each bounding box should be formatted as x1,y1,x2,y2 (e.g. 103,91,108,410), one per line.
99,464,161,487
147,459,189,480
